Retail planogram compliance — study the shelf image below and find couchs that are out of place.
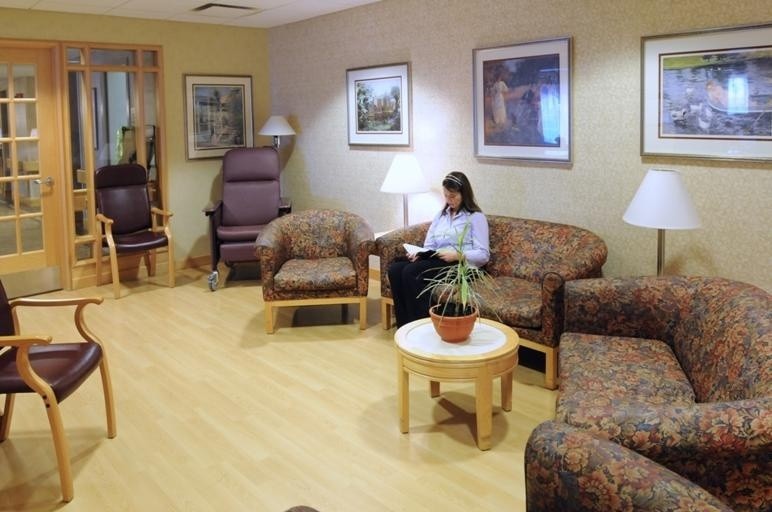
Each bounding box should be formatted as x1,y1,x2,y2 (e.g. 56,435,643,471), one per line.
254,206,380,334
277,421,739,512
376,211,607,390
553,275,771,510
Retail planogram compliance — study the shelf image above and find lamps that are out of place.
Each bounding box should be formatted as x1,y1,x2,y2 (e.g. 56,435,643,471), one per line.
622,168,702,274
257,113,297,147
384,154,427,228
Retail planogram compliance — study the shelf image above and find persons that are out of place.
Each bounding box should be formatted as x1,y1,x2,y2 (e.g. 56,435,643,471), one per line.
490,75,510,125
388,172,490,328
706,81,759,112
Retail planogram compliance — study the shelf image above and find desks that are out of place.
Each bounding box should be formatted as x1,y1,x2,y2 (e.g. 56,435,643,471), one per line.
393,316,522,451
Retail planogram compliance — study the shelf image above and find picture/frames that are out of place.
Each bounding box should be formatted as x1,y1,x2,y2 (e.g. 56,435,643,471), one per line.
640,22,772,169
472,36,575,170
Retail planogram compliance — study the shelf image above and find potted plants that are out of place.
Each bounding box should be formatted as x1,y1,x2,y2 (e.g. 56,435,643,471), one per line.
423,219,506,343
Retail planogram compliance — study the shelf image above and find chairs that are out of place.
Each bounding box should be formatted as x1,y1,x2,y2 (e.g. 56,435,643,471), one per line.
0,274,119,503
184,70,252,164
90,167,178,299
203,147,288,292
342,59,413,152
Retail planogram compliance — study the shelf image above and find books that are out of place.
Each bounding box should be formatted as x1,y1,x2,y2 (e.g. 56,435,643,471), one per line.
404,244,436,263
